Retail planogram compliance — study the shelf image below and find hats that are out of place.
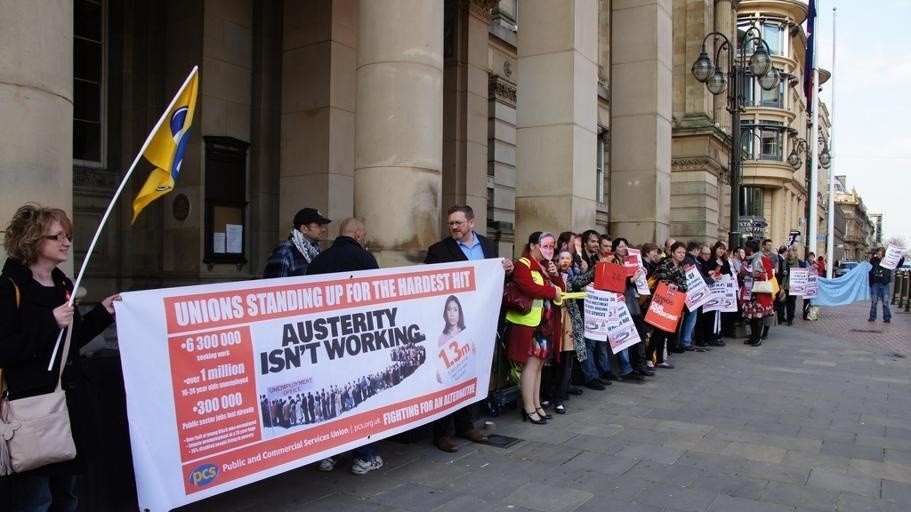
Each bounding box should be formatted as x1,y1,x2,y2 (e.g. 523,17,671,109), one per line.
293,207,332,223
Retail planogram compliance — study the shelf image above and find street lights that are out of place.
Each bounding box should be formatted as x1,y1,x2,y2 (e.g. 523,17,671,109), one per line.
691,27,780,254
785,129,830,263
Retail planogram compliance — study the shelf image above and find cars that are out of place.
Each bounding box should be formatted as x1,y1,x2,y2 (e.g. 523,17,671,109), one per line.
835,261,860,279
897,261,911,272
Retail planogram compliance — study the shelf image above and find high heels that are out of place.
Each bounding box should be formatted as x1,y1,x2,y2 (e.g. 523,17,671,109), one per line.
521,405,552,424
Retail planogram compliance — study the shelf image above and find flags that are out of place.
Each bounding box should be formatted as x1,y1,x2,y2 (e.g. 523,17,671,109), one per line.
131,67,203,228
809,260,871,307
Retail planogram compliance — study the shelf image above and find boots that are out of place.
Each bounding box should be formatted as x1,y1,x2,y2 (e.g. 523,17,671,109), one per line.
762,325,769,340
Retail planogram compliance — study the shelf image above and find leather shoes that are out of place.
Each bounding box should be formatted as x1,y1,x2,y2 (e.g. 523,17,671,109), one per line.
433,435,457,451
585,363,674,389
456,430,488,443
569,386,582,395
553,402,566,414
744,335,761,346
541,400,552,408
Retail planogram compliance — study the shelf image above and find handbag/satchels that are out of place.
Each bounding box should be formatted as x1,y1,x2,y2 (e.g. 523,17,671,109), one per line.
594,262,628,293
0,388,77,475
750,274,787,301
504,271,544,327
806,305,819,320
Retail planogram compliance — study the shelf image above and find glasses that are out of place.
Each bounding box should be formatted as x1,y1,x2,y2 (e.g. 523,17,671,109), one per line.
43,234,69,241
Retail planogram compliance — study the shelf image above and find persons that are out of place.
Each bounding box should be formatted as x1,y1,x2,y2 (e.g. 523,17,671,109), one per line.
434,295,477,386
263,207,333,278
303,216,386,473
413,202,516,454
863,246,907,323
506,228,829,423
253,341,426,430
0,203,123,511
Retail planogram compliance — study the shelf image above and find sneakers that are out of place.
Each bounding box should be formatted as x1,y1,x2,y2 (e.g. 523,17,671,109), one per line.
319,458,337,472
352,455,384,476
